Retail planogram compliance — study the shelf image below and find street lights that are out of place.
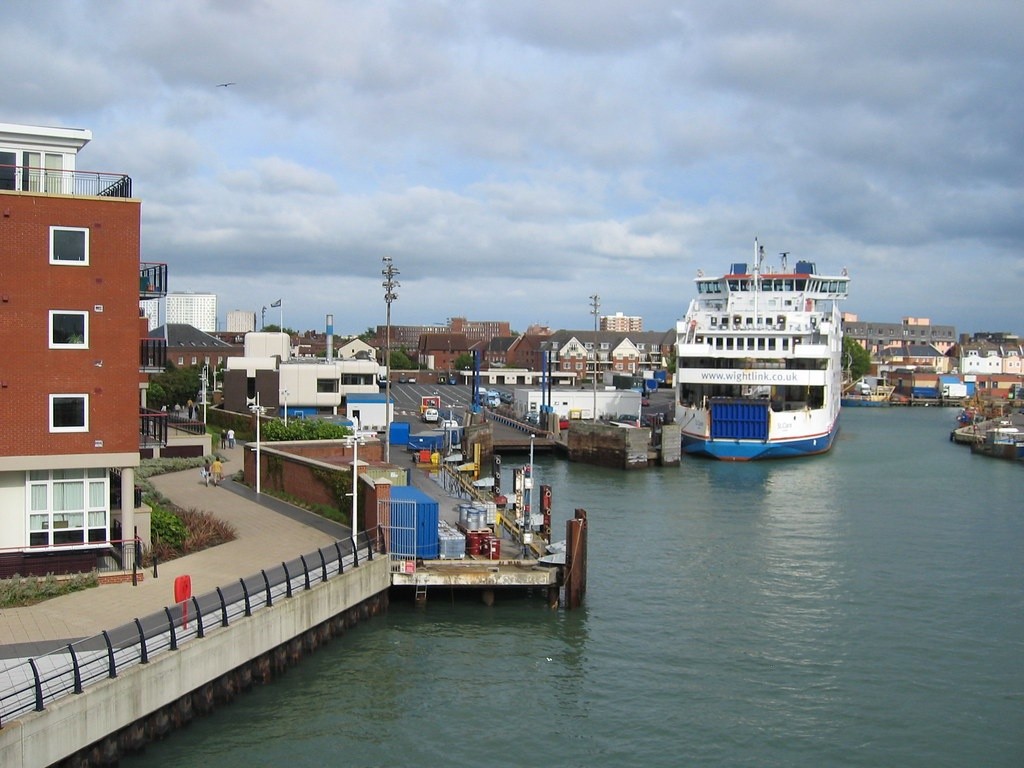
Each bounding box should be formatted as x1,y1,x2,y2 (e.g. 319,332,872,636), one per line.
587,292,601,424
382,255,401,465
246,390,267,493
528,434,536,532
342,418,365,556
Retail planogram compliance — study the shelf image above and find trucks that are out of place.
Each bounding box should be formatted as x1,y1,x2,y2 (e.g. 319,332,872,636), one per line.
418,395,441,424
864,375,888,394
941,384,967,399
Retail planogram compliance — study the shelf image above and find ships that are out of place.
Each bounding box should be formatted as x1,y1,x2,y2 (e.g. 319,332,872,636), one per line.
673,236,851,461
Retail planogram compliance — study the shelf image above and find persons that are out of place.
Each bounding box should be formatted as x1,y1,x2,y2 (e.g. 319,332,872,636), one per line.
202,428,237,489
162,398,200,420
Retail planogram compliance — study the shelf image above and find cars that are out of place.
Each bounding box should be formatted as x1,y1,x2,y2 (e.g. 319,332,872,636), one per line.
398,375,418,384
641,397,650,406
618,411,677,427
438,420,459,429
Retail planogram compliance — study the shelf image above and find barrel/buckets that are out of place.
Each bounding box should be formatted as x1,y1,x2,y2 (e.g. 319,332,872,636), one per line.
459,503,487,530
470,533,500,559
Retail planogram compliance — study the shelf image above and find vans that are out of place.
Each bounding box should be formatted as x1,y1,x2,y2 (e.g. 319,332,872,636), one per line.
911,386,941,399
474,386,512,409
855,381,872,396
